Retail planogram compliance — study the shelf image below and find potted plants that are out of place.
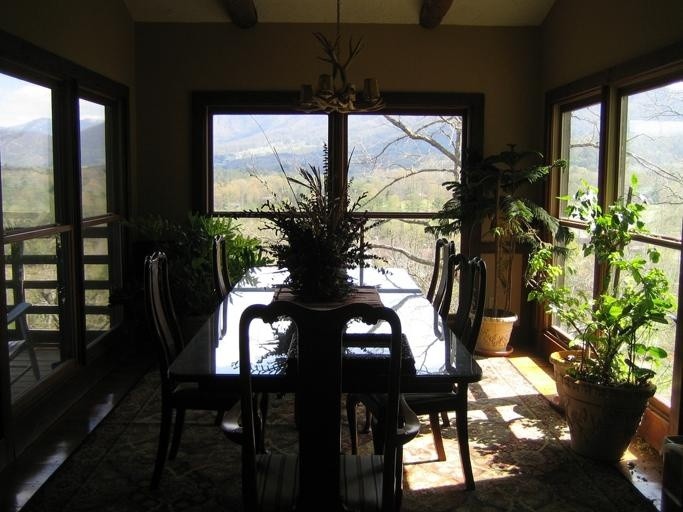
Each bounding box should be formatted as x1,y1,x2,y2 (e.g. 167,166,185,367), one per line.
546,170,675,467
424,143,568,358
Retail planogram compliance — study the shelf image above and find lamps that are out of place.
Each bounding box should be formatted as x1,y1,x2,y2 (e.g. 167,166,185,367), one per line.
290,0,384,114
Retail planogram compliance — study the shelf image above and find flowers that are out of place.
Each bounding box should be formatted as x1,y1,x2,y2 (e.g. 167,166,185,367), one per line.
256,162,383,305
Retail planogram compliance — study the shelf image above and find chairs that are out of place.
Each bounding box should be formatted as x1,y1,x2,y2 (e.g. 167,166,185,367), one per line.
237,301,402,512
148,252,269,479
346,253,489,492
425,238,456,318
212,234,232,298
4,227,43,405
